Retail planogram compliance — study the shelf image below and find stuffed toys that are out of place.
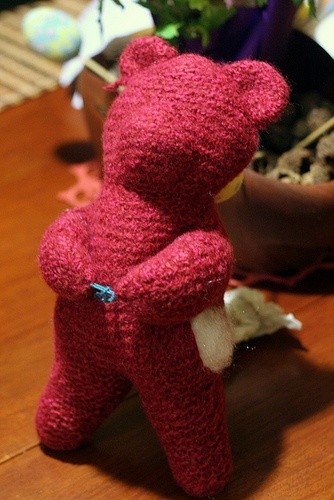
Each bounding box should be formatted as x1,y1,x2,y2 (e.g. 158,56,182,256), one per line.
35,37,288,495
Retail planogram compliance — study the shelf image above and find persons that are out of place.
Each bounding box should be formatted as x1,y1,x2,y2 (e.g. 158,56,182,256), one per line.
217,168,334,273
